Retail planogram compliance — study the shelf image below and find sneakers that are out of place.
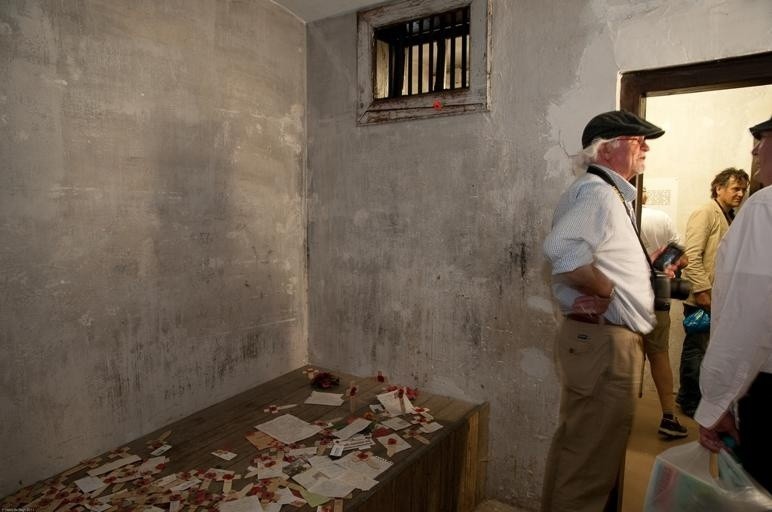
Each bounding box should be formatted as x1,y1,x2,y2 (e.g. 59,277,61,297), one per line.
658,412,688,437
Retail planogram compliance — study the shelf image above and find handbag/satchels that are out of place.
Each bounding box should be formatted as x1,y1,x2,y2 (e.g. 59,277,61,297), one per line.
645,434,772,512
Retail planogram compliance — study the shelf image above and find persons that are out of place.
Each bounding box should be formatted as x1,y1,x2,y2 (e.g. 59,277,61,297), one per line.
693,118,772,495
674,168,748,418
641,186,687,436
542,111,689,512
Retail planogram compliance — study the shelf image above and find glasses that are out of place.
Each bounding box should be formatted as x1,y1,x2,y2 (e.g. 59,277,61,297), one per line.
617,136,645,146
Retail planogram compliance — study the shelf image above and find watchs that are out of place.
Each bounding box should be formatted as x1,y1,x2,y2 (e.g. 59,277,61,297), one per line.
595,288,616,302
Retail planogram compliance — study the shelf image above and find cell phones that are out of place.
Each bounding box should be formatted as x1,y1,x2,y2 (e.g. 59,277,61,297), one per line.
652,241,685,273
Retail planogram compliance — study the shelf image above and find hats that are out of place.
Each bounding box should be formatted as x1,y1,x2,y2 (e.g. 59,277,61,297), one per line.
749,117,772,137
581,109,666,149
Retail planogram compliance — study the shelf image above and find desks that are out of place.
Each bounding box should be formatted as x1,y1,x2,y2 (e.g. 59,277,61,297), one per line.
1,360,492,512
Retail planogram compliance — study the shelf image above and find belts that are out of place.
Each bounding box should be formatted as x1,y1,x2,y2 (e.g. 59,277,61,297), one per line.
570,311,611,325
